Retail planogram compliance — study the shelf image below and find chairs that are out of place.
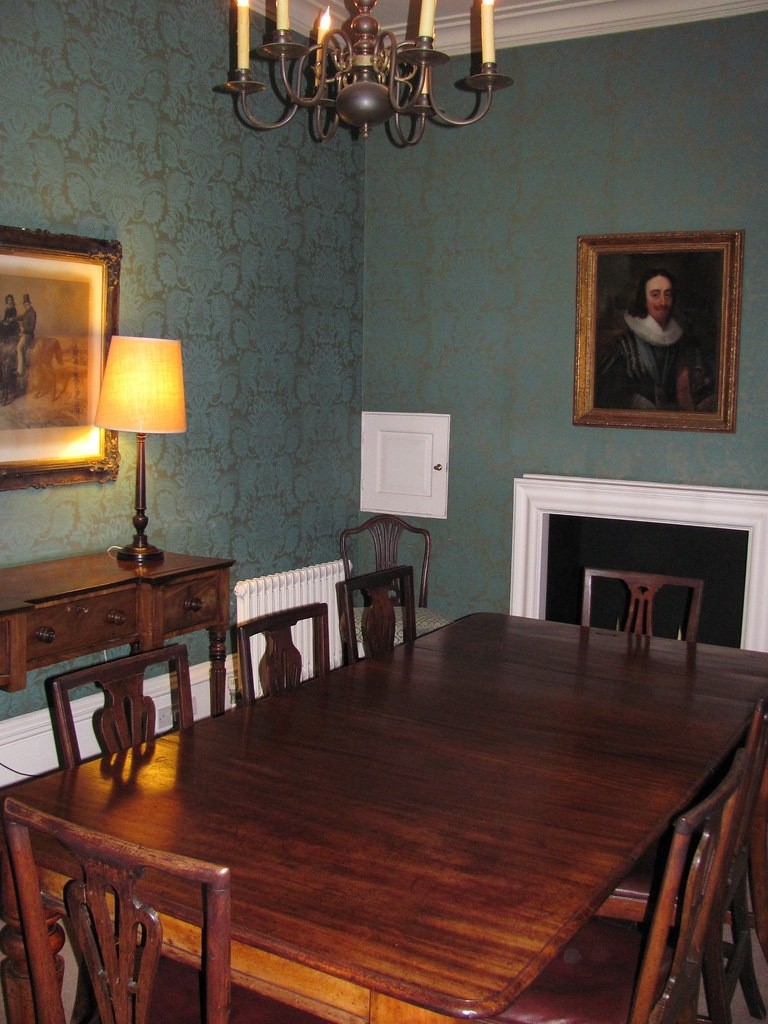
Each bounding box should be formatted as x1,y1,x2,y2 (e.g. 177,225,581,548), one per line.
230,601,331,703
42,644,194,767
336,512,451,642
581,567,704,642
3,797,233,1024
479,749,751,1024
590,694,768,1024
338,564,417,665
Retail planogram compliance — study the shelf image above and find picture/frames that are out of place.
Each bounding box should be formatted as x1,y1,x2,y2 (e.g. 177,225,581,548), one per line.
1,226,123,488
565,229,744,434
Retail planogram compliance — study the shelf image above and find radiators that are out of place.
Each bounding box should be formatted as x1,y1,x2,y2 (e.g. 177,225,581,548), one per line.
230,562,351,695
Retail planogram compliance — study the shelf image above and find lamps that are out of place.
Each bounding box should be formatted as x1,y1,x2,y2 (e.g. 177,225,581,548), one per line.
220,0,511,149
89,335,185,563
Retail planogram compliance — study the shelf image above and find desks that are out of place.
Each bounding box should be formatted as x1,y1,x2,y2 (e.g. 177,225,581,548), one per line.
0,549,235,729
0,613,768,1024
